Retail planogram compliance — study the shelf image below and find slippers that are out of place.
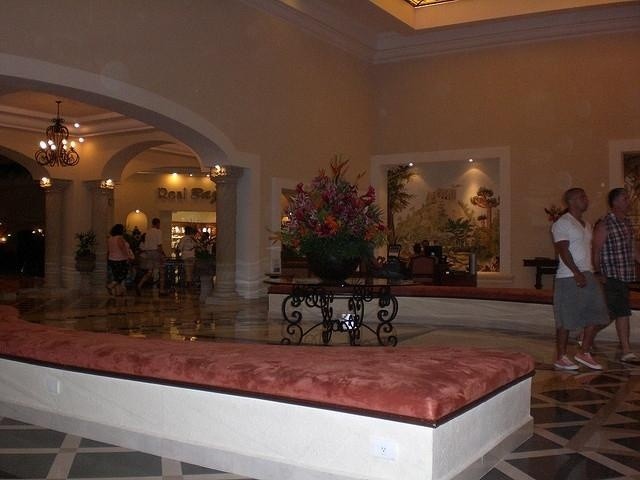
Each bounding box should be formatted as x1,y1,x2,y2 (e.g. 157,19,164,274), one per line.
620,353,640,362
576,335,592,354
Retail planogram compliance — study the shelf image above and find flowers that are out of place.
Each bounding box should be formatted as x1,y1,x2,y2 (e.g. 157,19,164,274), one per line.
267,151,395,268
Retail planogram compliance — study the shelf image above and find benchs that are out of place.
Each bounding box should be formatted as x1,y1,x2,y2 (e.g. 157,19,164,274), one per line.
268,279,640,345
1,304,537,480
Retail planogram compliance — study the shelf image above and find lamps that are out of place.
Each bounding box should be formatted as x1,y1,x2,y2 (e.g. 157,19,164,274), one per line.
99,177,115,207
34,100,81,168
208,163,230,184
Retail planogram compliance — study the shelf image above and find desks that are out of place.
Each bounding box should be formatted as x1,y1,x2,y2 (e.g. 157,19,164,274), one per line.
260,275,422,347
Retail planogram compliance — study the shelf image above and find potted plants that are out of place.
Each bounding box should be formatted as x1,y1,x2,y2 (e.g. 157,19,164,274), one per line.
73,229,100,272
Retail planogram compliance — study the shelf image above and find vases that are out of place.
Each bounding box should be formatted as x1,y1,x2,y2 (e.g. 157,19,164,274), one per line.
305,257,360,287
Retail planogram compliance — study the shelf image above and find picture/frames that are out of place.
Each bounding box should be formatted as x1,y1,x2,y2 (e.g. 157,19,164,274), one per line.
369,144,513,284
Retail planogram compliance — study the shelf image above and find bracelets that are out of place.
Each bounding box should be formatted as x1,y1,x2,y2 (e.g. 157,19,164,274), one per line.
593,270,602,277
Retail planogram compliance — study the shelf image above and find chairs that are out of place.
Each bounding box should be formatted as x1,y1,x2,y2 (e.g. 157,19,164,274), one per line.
409,256,435,285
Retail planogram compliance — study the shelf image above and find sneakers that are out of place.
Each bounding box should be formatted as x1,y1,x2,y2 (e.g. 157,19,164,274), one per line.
574,351,602,369
554,356,579,370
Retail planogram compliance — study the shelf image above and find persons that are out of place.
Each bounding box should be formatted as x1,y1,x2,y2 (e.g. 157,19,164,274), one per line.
421,239,429,252
550,187,610,372
103,218,217,297
407,244,422,271
578,187,640,363
358,240,386,278
